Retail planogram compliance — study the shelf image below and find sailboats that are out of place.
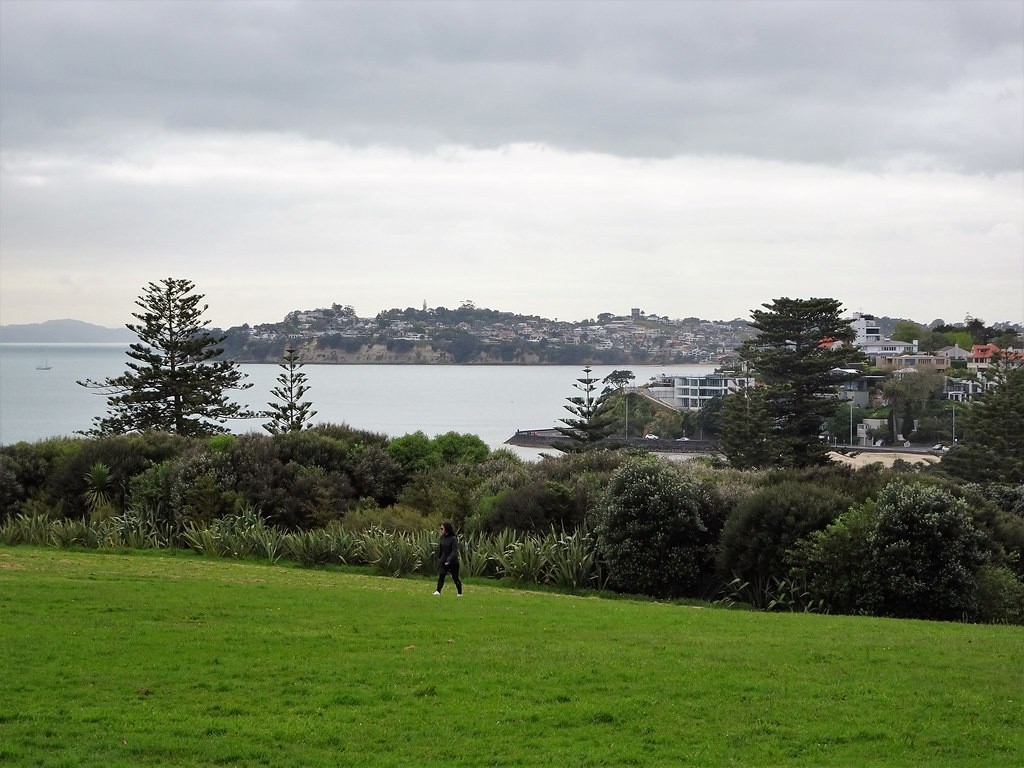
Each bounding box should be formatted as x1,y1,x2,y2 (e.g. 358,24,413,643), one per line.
36,352,52,370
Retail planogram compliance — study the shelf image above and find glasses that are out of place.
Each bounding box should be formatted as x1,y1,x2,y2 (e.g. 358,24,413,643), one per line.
440,527,444,531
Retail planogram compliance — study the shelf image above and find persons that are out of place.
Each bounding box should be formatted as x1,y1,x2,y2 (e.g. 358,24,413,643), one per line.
433,522,463,597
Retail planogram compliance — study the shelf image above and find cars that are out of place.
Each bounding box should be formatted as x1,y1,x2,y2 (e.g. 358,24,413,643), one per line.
933,443,944,450
645,433,660,441
675,437,690,442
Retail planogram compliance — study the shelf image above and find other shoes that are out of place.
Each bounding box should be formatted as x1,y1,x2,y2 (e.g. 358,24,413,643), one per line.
457,594,463,597
432,591,440,596
